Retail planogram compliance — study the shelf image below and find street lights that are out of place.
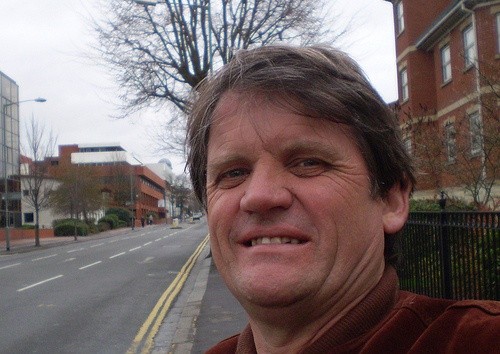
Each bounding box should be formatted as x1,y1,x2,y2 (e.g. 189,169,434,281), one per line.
3,98,47,251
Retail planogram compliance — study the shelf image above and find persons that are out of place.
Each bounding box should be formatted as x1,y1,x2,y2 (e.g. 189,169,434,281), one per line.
184,45,500,354
141,215,152,228
